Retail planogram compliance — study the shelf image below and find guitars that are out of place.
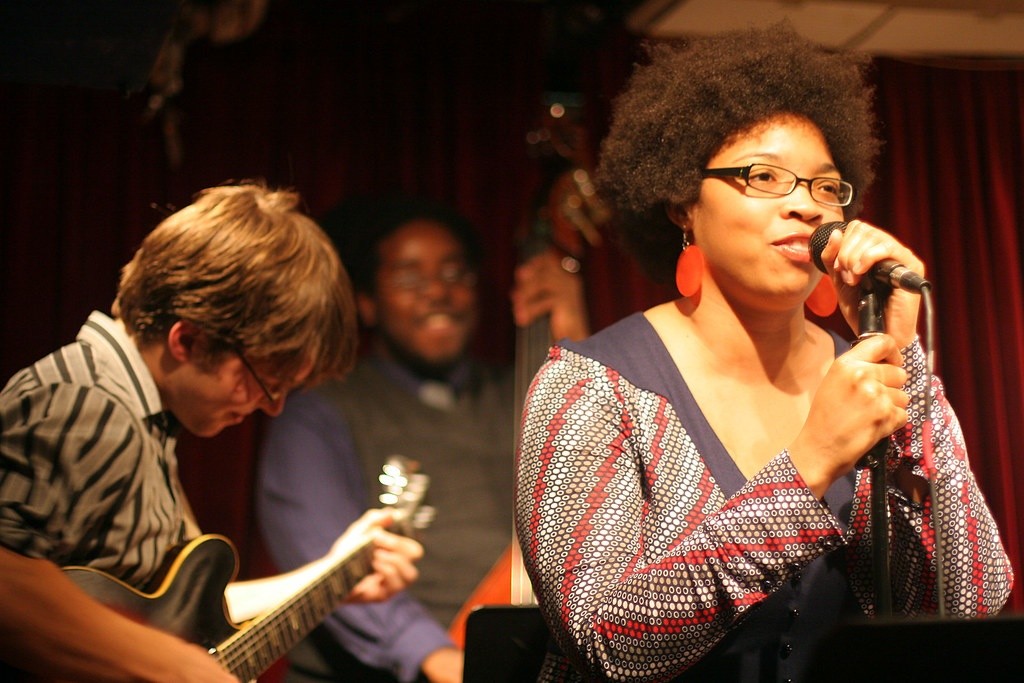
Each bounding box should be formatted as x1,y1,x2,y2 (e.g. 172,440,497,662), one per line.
63,454,432,682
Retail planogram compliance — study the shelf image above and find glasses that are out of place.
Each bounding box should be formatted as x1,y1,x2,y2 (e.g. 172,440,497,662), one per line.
223,340,304,412
700,163,854,206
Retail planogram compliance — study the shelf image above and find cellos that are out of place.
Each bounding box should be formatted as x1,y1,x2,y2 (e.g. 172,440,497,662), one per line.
446,100,602,654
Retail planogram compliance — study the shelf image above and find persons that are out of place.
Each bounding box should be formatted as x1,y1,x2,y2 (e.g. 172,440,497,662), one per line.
0,185,425,683
245,210,589,682
515,21,1014,682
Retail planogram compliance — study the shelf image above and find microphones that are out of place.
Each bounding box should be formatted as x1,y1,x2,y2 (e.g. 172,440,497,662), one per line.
808,221,932,296
